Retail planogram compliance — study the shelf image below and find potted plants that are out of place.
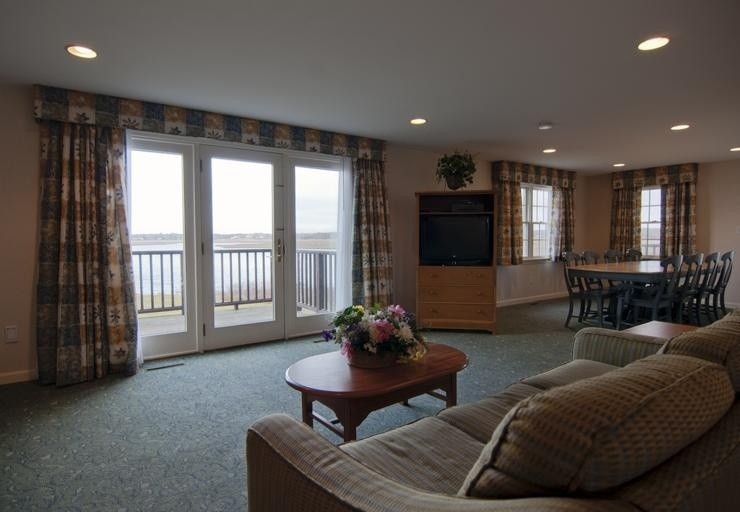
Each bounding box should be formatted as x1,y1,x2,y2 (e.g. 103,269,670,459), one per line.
435,150,476,190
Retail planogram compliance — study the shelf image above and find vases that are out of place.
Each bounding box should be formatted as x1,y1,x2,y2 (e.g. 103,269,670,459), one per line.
345,349,390,367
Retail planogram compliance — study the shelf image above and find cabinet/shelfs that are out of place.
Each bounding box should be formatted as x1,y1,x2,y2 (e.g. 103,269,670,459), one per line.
414,190,499,335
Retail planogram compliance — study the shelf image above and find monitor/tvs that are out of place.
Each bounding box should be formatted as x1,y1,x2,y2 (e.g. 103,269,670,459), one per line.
419,214,494,266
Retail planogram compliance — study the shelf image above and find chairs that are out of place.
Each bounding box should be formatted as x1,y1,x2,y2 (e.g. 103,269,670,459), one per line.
563,249,734,328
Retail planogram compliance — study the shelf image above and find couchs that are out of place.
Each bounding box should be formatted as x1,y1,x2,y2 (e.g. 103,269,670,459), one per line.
243,310,739,512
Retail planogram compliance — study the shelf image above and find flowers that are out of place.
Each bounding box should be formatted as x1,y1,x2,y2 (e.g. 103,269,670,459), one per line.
322,305,427,364
